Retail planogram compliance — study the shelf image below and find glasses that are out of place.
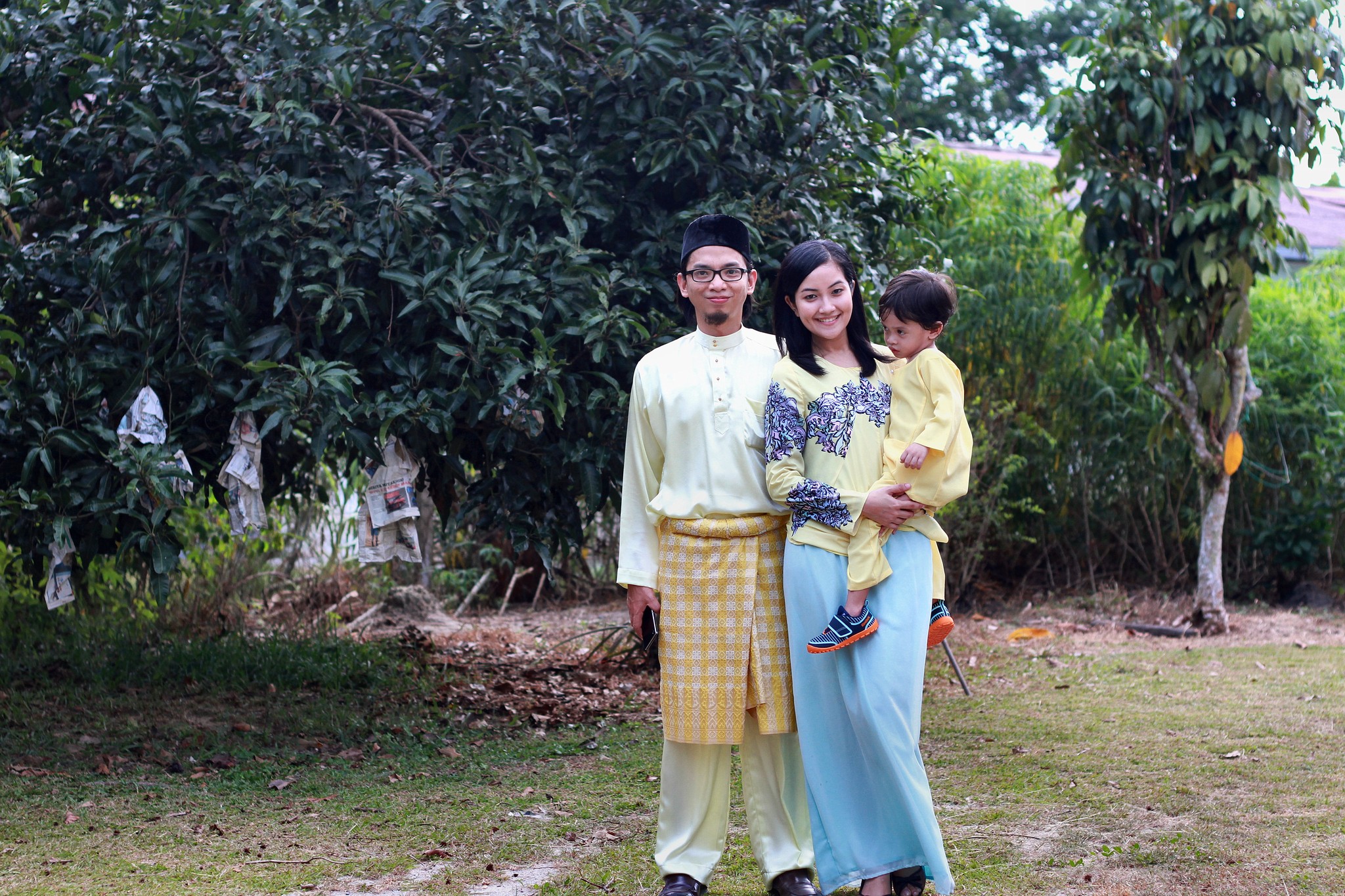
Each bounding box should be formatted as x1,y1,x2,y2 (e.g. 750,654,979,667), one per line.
685,268,748,283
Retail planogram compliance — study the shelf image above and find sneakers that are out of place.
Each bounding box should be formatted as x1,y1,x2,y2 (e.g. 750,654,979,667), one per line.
926,599,953,646
808,599,878,655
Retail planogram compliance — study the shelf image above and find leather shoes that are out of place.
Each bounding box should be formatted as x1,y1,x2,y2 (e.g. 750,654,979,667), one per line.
771,868,823,896
657,873,708,896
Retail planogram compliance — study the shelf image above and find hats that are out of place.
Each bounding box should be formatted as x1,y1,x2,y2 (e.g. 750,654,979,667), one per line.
681,214,751,271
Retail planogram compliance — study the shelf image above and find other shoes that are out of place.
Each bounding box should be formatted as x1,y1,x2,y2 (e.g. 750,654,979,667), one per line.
892,871,925,896
858,875,892,896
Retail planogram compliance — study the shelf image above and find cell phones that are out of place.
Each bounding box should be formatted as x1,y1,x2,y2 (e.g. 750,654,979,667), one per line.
641,604,658,652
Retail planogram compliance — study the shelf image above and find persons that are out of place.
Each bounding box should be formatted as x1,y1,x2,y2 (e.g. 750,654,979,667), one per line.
803,268,975,654
612,213,829,896
758,236,955,896
